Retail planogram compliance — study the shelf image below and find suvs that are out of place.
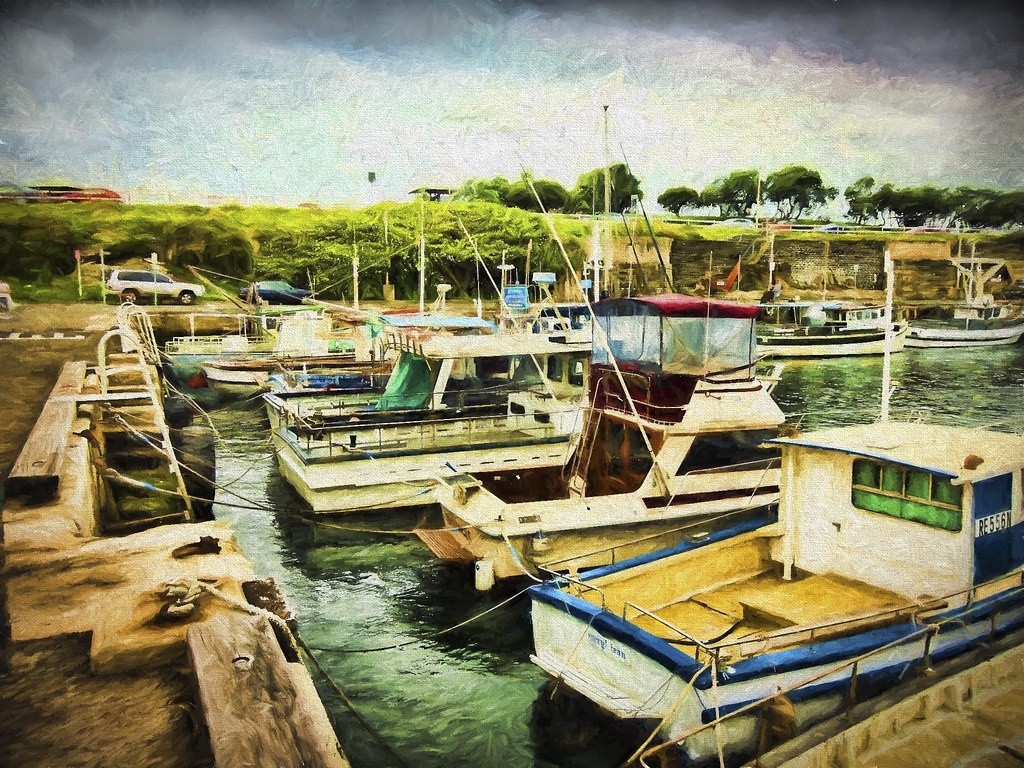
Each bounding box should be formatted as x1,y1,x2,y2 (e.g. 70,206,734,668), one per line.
106,269,206,306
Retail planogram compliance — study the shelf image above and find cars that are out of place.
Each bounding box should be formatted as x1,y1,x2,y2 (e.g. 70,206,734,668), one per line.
237,280,313,305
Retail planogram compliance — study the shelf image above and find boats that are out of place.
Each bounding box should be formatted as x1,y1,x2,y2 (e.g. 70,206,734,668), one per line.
756,234,909,356
411,161,786,592
905,241,1024,347
528,249,1024,768
261,216,787,515
164,231,601,393
